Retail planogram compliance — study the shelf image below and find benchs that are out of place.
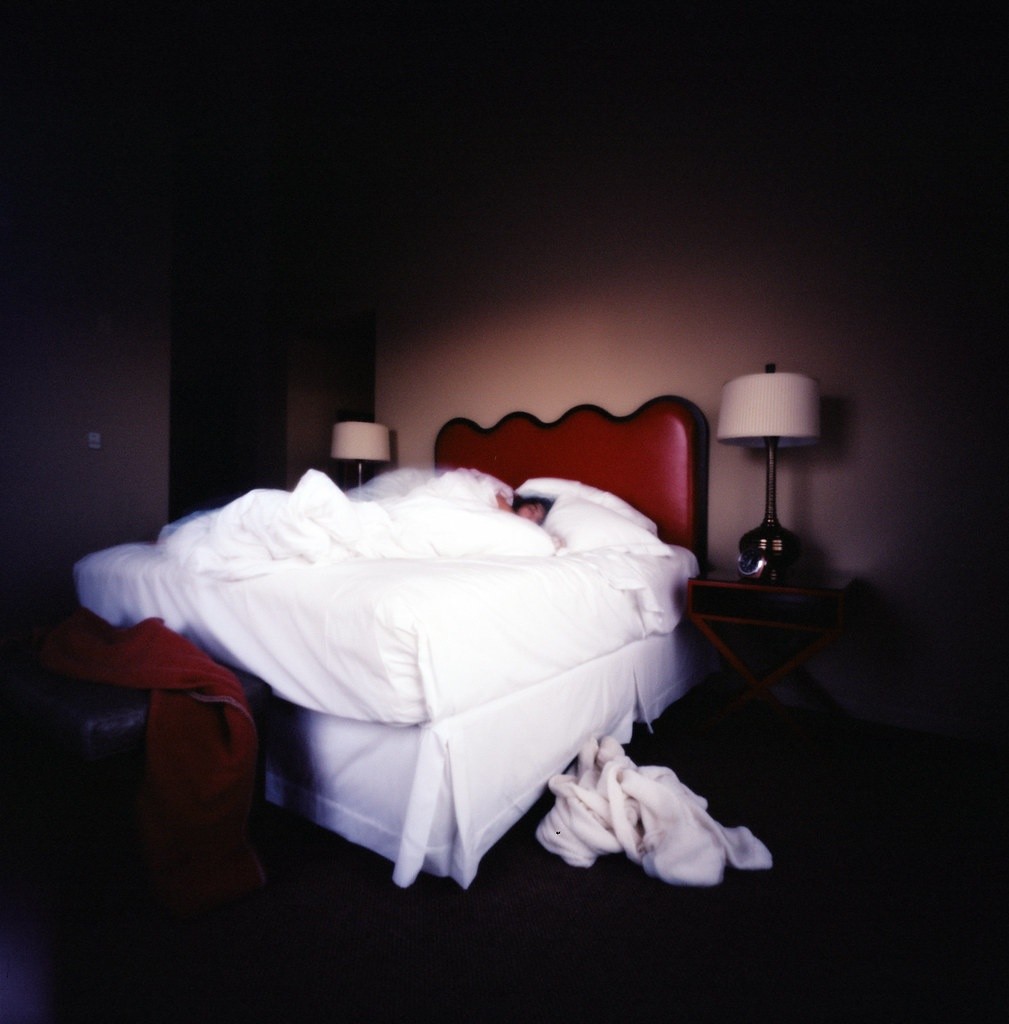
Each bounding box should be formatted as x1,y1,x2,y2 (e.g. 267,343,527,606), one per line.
0,617,269,924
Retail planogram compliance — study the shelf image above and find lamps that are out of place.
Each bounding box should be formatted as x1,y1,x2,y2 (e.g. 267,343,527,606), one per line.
330,421,391,486
717,363,820,554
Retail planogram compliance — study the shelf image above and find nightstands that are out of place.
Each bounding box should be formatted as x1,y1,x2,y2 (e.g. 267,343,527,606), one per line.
688,554,864,743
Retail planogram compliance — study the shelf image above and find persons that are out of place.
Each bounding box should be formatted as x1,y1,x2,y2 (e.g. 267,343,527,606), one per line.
470,468,553,526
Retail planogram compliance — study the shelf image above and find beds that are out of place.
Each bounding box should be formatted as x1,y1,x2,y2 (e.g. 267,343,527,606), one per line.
73,395,710,891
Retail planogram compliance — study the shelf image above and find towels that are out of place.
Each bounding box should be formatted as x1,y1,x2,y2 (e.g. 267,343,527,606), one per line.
37,603,260,926
534,733,774,888
